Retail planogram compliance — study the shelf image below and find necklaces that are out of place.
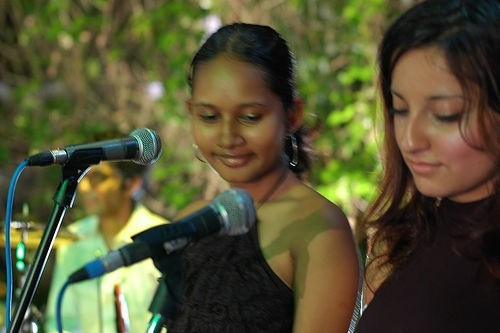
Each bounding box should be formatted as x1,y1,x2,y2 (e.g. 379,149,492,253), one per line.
255,162,289,211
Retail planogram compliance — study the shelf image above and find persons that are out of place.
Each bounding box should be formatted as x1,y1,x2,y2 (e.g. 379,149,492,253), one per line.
166,22,360,333
44,162,172,333
353,0,500,333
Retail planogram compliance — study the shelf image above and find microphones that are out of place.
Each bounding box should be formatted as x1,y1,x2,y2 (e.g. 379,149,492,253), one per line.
71,188,256,283
28,128,161,166
